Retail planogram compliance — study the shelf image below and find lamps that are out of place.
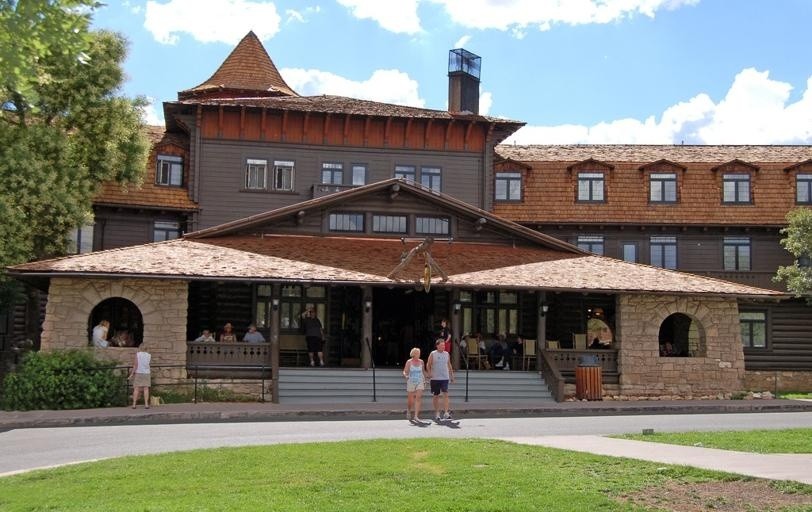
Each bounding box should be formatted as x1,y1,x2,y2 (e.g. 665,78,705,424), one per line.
271,295,280,311
453,296,461,314
593,307,603,316
364,295,372,312
539,301,549,316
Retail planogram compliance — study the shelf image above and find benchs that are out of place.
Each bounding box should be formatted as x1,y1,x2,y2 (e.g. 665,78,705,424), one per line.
278,334,310,365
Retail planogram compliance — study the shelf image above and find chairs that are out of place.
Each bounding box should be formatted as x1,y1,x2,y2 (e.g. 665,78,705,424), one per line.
464,337,489,371
522,339,537,371
544,340,561,352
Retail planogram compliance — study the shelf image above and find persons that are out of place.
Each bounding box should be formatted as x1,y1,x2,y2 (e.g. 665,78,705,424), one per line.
195,329,216,343
425,339,455,420
111,330,136,347
437,319,682,371
302,308,326,367
401,347,427,422
242,324,267,343
126,342,151,408
91,320,111,347
221,322,236,343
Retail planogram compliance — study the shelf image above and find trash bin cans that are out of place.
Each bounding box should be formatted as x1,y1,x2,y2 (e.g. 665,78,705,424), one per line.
575,363,603,402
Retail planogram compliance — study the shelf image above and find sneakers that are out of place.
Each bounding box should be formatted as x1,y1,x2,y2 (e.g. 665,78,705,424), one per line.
495,360,503,367
407,409,412,420
443,411,452,419
433,413,440,420
414,416,421,422
502,366,510,370
